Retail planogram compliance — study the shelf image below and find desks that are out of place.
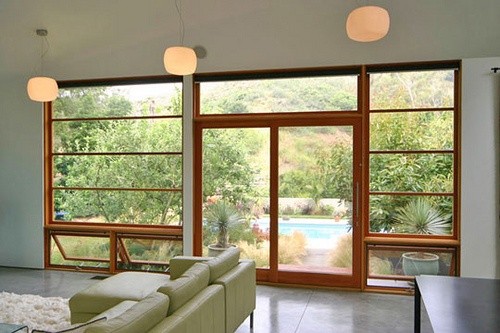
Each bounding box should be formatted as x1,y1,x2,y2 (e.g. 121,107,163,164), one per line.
413,274,500,333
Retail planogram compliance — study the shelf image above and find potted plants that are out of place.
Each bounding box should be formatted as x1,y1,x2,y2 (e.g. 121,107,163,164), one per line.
391,198,452,276
203,200,250,257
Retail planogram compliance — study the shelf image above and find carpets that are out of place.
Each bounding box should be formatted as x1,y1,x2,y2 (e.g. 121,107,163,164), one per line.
0,291,72,333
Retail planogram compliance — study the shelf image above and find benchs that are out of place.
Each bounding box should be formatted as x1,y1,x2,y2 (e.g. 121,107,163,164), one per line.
0,248,256,333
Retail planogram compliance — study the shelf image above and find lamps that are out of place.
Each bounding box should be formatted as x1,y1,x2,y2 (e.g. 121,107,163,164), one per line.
26,29,58,101
163,0,197,75
346,5,390,42
